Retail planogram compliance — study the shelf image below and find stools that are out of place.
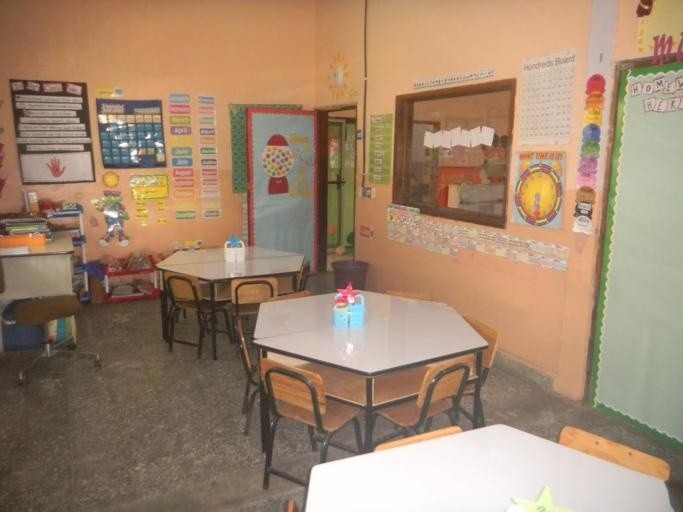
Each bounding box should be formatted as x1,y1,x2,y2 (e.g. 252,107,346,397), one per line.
4,295,102,384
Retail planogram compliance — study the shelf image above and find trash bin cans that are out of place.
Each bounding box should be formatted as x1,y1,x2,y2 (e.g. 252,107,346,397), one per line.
330,260,369,290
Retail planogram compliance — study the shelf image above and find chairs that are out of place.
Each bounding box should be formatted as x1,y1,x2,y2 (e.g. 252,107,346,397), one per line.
424,313,499,432
156,247,311,363
371,425,463,453
256,358,363,490
369,352,477,453
557,424,669,488
236,315,262,437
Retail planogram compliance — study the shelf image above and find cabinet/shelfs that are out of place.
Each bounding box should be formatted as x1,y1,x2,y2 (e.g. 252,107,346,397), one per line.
1,203,92,307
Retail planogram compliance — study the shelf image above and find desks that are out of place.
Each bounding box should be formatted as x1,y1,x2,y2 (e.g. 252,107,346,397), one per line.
252,289,492,453
302,422,675,511
1,233,74,299
